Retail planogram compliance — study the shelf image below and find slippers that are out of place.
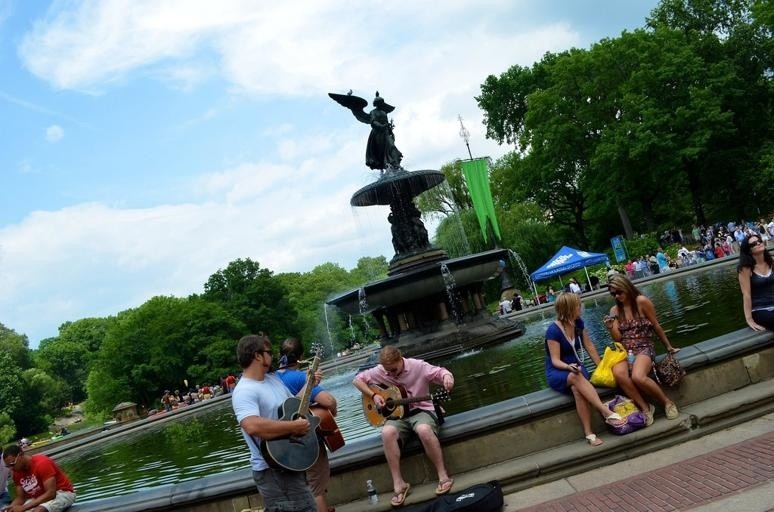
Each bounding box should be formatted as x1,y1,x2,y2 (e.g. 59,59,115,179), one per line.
436,479,454,495
602,411,626,428
585,433,603,447
391,483,410,506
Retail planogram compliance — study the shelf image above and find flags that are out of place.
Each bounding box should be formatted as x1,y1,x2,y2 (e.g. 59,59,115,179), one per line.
459,156,503,244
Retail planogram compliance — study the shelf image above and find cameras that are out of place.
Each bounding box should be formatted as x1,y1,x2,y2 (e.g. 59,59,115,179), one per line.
608,316,615,321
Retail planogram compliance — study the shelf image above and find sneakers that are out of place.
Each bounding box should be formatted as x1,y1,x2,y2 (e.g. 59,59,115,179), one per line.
644,402,656,427
664,400,679,420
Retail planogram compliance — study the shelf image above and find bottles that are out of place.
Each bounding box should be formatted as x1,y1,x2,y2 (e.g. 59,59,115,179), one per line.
627,349,635,374
365,480,380,506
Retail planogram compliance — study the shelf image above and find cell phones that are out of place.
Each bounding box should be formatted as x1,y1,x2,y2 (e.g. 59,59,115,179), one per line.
571,365,578,370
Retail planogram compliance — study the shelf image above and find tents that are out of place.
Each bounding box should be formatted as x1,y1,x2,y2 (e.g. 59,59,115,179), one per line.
530,243,609,304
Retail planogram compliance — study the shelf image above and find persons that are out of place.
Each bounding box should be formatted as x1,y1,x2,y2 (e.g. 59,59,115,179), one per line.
738,234,773,333
497,279,584,317
230,335,335,510
582,249,674,292
353,345,456,505
542,272,681,446
672,213,773,269
363,89,404,171
161,372,241,412
1,445,76,511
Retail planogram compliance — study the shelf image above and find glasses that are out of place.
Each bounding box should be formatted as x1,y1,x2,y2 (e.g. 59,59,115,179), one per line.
5,453,19,467
263,350,273,356
610,290,622,296
750,239,762,247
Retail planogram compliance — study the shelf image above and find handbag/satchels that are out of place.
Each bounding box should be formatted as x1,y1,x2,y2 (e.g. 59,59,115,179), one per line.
590,342,627,388
654,351,686,387
601,395,648,435
